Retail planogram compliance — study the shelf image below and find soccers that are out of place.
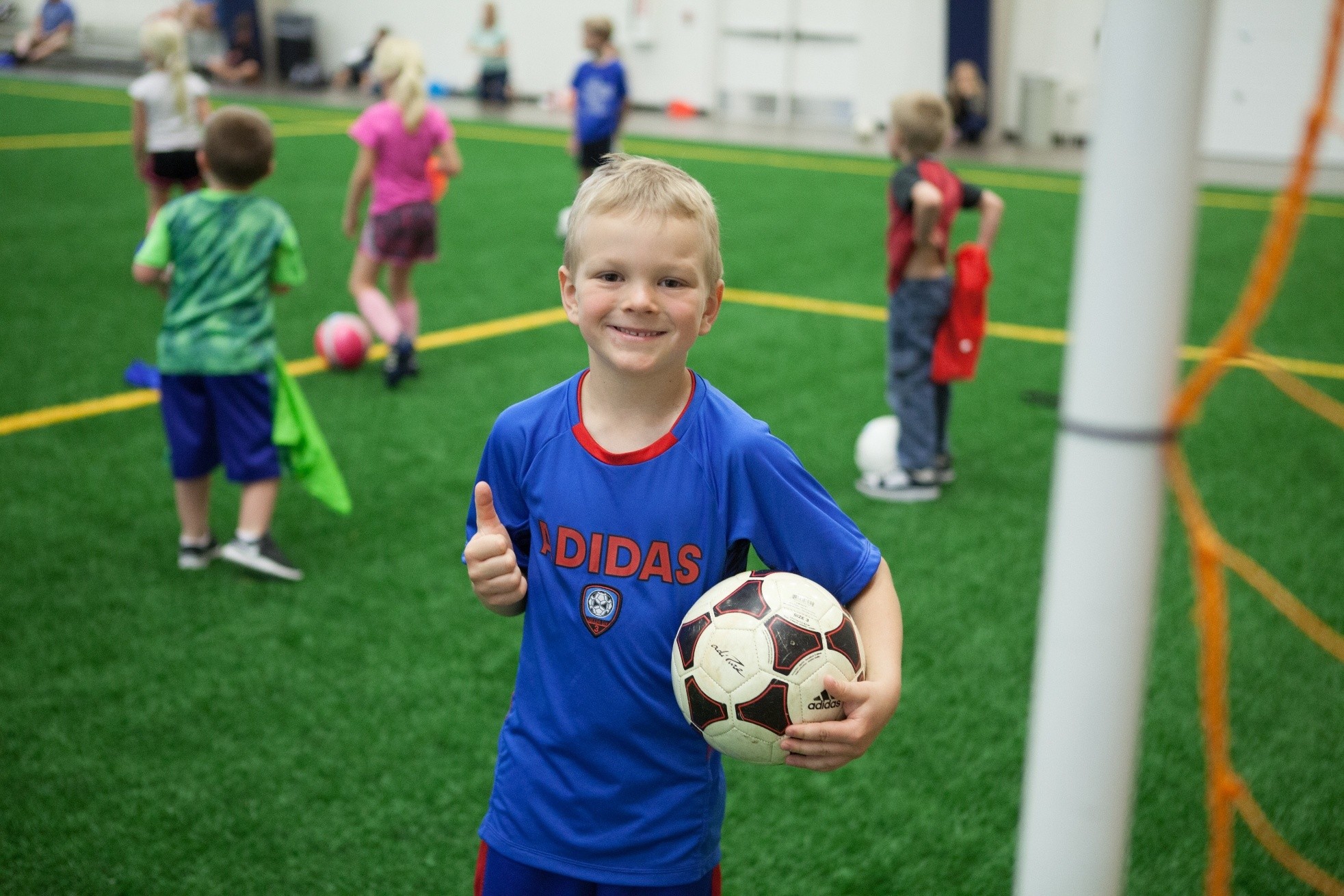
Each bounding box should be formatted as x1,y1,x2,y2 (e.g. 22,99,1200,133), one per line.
314,313,370,367
671,570,871,762
854,416,901,470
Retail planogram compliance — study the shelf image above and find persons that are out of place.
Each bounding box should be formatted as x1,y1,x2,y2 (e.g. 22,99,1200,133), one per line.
129,101,309,581
563,14,628,184
461,149,905,895
339,35,461,383
126,15,214,293
856,95,1006,501
0,0,519,122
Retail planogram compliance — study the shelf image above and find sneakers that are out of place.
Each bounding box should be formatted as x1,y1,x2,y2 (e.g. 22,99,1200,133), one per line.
177,532,216,570
853,465,941,502
384,332,413,385
220,535,304,582
